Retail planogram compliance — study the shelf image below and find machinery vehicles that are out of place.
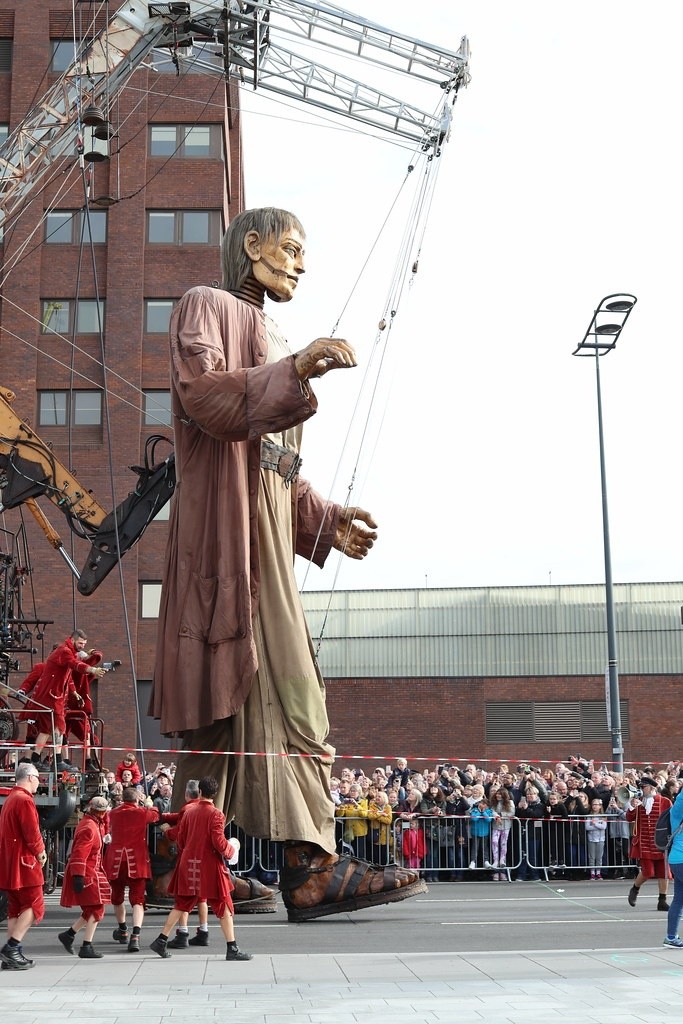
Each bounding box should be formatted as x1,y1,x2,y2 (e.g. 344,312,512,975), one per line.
0,0,471,880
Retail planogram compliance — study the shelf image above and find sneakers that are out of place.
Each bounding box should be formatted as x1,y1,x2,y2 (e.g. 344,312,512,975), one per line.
11,757,101,772
58,932,76,955
127,934,141,952
112,929,129,944
227,946,253,959
656,902,671,911
627,887,639,907
189,930,210,945
415,857,639,882
0,943,35,971
78,945,105,958
663,935,683,949
167,933,189,948
150,939,171,958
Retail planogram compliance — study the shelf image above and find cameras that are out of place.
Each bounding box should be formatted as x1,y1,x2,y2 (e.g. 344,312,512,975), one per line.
584,778,588,782
524,771,530,775
453,789,457,794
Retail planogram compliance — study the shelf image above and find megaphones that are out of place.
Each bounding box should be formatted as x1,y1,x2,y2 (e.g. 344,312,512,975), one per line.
617,787,643,804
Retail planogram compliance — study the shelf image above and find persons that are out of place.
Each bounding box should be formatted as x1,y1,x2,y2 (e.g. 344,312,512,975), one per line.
147,206,428,922
331,756,683,881
0,763,48,970
626,777,672,911
104,787,158,951
17,630,109,772
150,777,253,960
158,780,208,947
103,754,176,814
663,784,683,948
58,797,112,958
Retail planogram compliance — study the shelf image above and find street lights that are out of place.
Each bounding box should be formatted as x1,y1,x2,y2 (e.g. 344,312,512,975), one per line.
573,293,641,773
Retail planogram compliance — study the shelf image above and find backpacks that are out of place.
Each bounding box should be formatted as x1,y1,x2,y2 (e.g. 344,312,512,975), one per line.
654,794,683,852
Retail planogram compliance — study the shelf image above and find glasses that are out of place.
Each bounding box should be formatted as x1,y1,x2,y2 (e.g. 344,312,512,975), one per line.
337,765,637,809
641,786,647,789
27,773,39,779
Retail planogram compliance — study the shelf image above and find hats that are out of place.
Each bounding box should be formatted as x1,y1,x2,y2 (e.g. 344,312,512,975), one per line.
638,778,658,786
91,797,108,811
157,773,167,778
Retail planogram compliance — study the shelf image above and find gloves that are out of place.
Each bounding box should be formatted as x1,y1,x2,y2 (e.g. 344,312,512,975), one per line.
73,875,84,893
36,851,48,869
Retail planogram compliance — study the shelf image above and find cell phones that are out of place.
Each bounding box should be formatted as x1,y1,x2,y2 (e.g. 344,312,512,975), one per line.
395,780,399,787
355,768,360,773
344,799,352,803
385,766,391,774
610,797,615,801
568,757,571,764
521,797,526,803
